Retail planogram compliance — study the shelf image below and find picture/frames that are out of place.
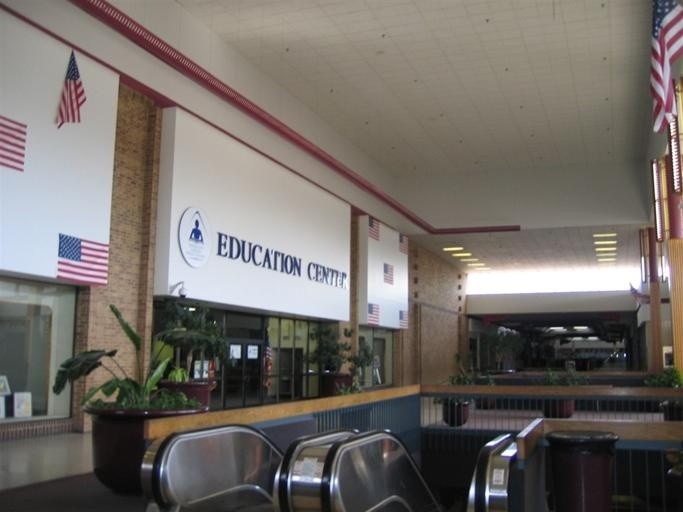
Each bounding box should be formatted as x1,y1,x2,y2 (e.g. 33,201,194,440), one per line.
661,346,675,368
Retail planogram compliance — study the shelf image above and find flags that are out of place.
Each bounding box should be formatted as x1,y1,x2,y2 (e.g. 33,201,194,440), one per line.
261,328,273,393
367,215,410,330
56,232,110,286
630,288,651,304
0,115,29,173
56,50,87,130
647,0,683,134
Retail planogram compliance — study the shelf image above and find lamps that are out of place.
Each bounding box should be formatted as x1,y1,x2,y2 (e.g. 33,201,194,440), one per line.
168,280,186,298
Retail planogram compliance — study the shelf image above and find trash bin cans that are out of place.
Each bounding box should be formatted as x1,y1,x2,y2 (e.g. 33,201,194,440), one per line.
545,431,619,510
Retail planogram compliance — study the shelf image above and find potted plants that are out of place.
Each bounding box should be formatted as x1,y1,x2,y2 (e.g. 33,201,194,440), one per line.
641,367,682,420
305,323,372,397
530,368,585,417
434,351,498,425
52,301,229,495
